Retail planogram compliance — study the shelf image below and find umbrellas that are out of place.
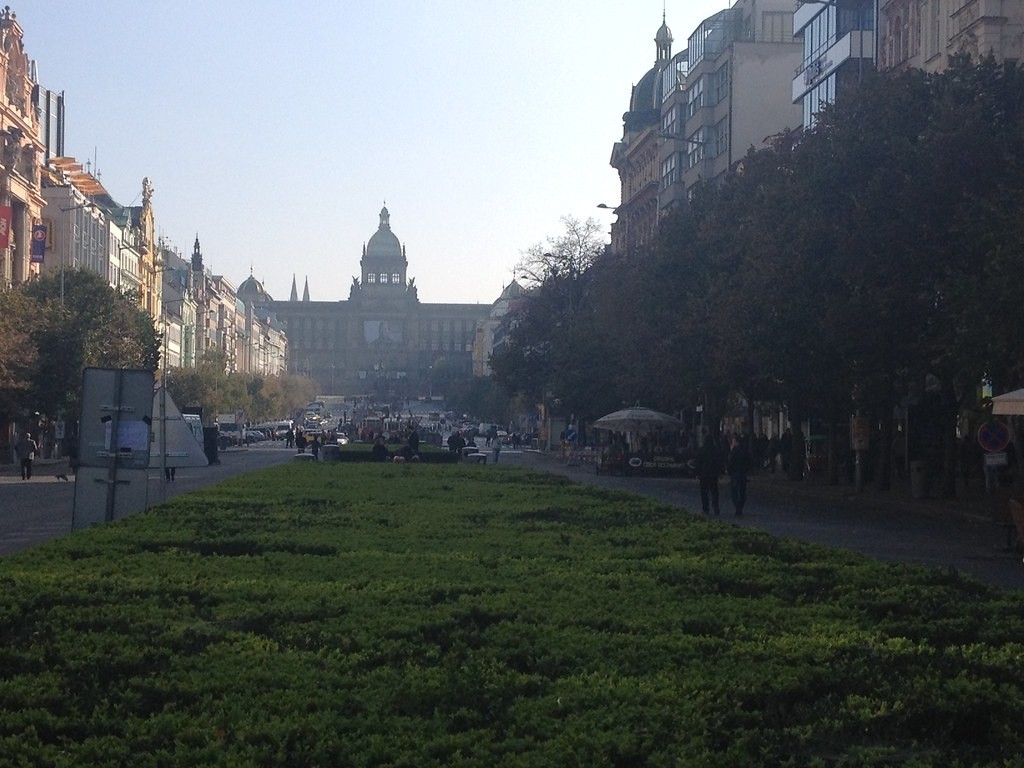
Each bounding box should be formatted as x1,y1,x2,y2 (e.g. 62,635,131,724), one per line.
591,400,682,453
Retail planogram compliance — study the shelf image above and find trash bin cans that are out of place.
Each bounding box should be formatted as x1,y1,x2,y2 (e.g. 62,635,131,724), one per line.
321,444,339,461
910,460,927,498
462,446,487,464
294,452,316,461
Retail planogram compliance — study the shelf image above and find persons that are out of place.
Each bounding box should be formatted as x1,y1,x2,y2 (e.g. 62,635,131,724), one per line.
285,426,531,465
12,433,37,481
165,467,175,482
559,425,806,516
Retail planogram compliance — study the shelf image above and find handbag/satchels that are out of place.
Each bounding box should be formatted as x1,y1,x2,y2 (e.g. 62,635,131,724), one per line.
29,452,34,459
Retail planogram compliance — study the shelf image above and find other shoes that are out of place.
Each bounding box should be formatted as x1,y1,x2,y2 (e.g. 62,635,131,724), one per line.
27,475,32,479
22,475,26,479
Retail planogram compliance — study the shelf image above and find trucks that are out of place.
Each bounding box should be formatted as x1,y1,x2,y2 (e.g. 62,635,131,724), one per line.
275,422,296,441
217,414,246,447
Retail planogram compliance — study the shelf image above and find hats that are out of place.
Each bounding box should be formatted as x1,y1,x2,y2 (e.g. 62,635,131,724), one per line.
25,433,31,437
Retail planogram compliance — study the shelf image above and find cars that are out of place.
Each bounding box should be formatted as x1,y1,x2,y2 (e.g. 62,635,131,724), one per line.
497,430,508,444
454,419,478,436
301,411,349,446
246,425,273,444
